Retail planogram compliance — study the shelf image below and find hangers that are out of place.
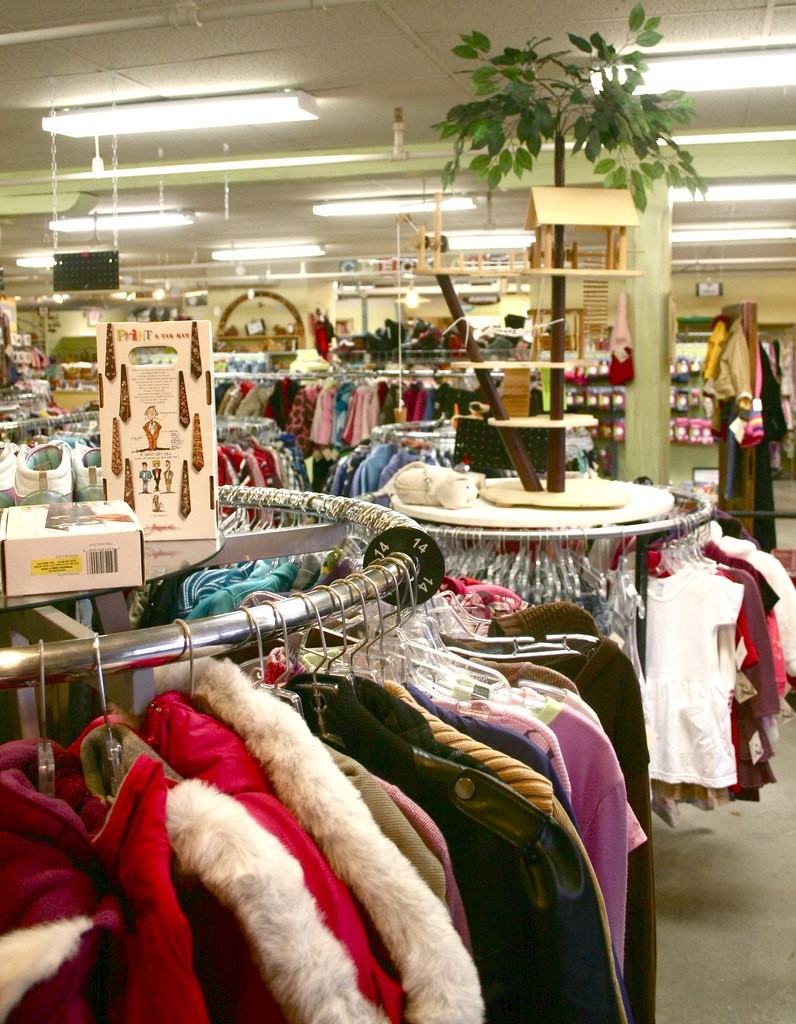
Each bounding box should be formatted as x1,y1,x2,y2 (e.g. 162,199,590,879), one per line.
353,472,734,623
0,482,608,852
0,308,616,472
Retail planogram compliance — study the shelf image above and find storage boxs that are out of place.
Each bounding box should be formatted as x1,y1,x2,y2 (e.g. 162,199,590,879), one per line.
1,501,151,598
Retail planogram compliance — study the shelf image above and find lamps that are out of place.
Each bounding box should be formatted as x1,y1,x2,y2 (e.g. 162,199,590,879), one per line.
41,88,323,139
395,258,430,308
211,241,327,262
48,210,197,234
312,194,478,219
425,191,538,254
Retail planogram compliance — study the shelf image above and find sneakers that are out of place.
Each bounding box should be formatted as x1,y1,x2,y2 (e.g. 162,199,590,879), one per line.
72,439,104,502
14,437,72,506
0,442,19,508
